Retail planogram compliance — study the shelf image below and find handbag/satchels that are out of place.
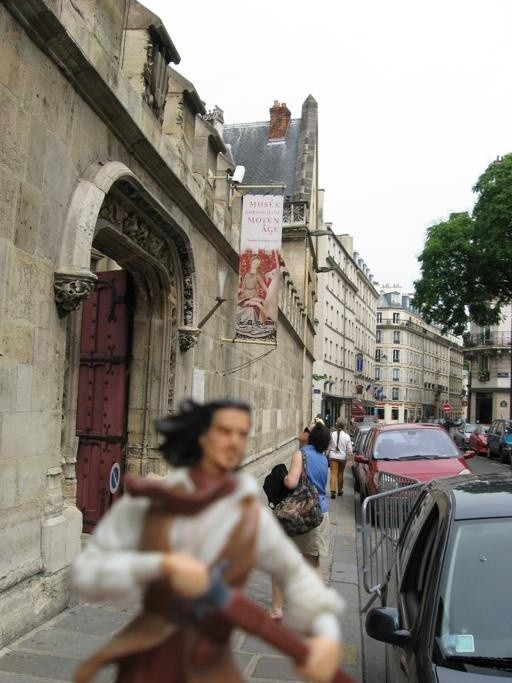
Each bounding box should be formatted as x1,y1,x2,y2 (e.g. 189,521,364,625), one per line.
275,485,323,537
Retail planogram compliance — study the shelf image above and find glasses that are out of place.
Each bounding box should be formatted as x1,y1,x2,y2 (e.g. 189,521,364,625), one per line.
304,427,311,435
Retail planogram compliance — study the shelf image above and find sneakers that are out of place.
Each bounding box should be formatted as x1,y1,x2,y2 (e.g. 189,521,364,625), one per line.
330,488,344,499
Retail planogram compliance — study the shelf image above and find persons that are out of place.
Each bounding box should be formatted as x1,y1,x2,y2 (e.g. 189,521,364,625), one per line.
64,396,349,682
239,254,272,319
307,412,357,498
265,420,330,624
405,412,465,437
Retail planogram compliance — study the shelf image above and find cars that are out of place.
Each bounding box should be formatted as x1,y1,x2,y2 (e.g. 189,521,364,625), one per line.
364,471,511,683
350,417,512,526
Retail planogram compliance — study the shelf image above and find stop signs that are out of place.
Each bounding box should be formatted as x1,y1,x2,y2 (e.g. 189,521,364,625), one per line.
442,403,452,413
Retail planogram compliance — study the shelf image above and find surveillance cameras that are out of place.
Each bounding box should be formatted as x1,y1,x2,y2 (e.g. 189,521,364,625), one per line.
231,165,246,184
